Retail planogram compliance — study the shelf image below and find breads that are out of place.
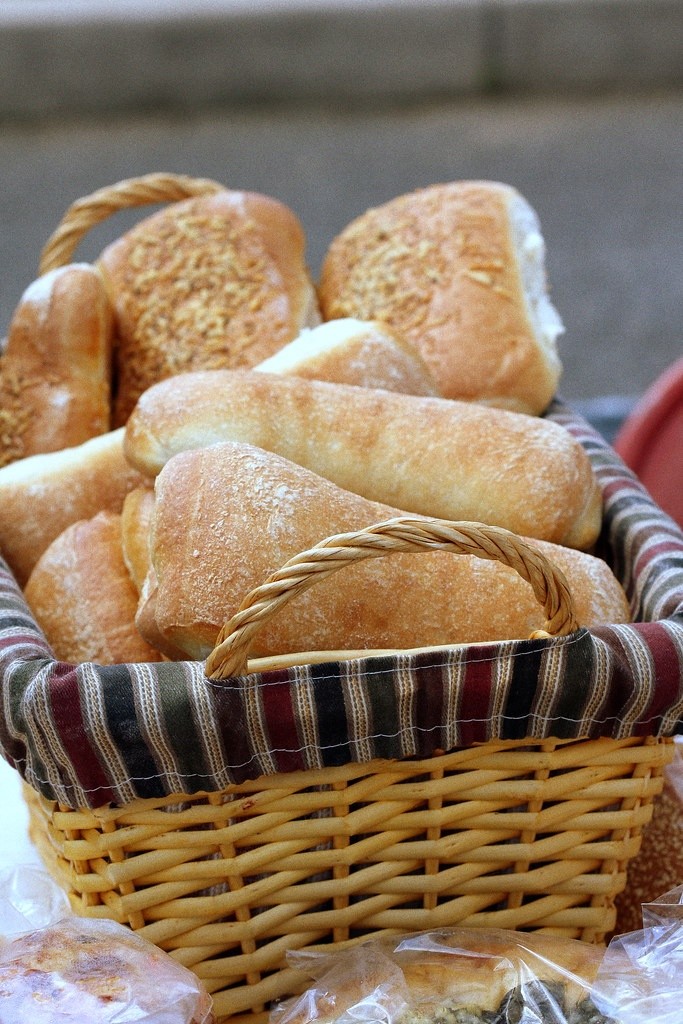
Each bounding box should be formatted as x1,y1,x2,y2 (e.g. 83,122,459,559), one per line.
0,178,633,666
0,736,683,1024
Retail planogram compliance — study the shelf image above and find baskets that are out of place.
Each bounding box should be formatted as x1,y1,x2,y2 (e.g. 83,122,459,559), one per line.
0,172,683,1023
